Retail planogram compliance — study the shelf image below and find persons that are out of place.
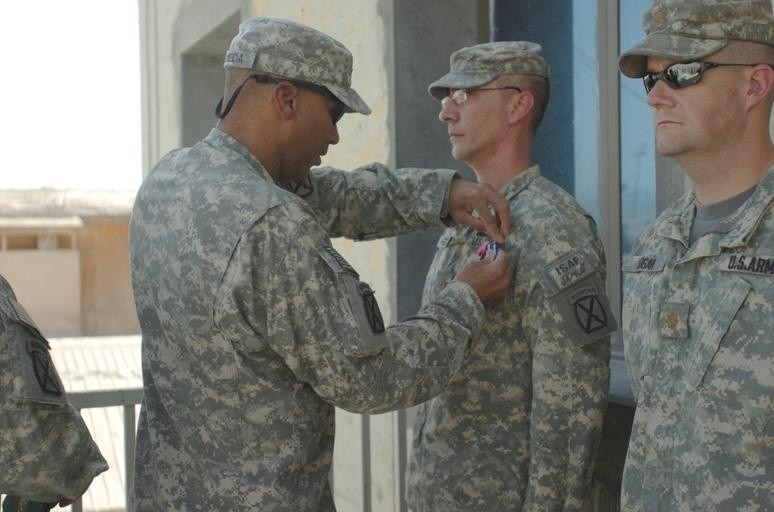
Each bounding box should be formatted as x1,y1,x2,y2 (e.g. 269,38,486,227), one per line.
0,276,108,512
404,40,619,512
128,17,514,512
619,0,774,512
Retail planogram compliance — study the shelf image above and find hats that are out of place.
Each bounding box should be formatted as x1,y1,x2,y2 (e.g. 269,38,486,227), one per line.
619,0,774,78
222,15,371,115
428,41,552,102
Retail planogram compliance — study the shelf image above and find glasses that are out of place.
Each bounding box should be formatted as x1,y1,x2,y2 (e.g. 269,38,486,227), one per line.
256,75,348,125
442,86,522,107
643,59,774,93
